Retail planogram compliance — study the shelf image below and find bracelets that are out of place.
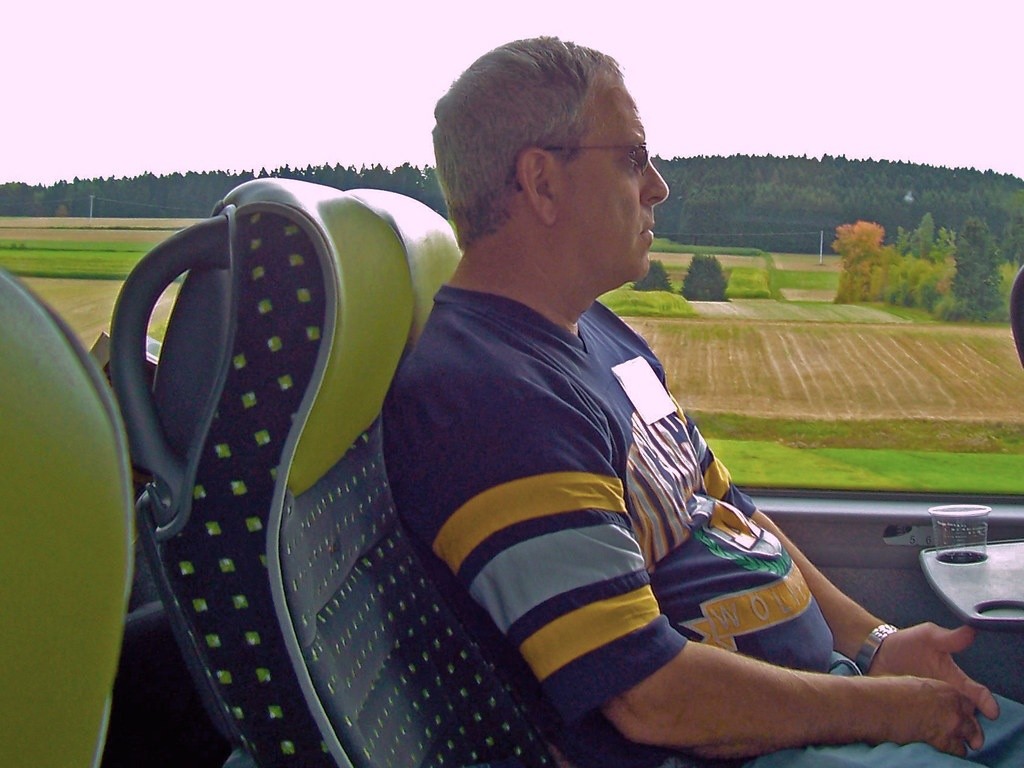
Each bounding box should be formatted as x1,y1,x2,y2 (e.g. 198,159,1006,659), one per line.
853,623,899,676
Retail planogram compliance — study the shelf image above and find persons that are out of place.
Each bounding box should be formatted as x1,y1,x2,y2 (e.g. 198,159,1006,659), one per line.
380,34,1024,768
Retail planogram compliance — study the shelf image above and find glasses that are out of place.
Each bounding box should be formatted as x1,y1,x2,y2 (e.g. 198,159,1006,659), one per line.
504,140,649,185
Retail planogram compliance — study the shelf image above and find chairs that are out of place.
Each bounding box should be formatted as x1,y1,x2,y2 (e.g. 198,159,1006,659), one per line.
104,174,576,768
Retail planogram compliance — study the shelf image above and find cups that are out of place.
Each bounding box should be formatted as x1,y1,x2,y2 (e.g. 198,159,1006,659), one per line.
927,503,992,565
